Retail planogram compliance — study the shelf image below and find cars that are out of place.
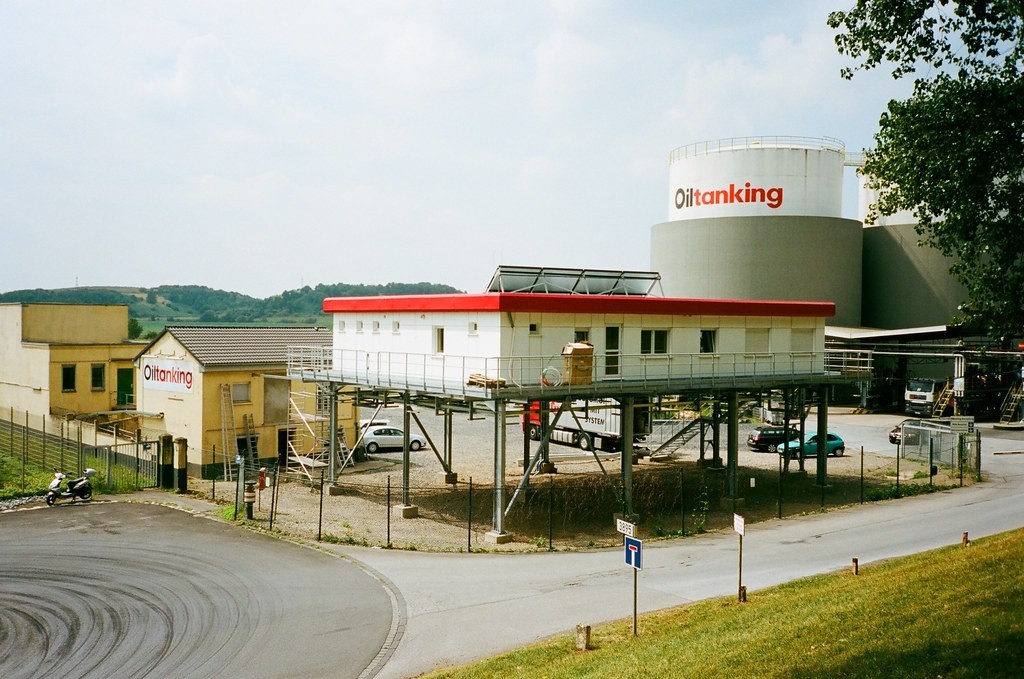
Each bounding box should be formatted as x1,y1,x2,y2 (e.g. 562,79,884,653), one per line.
777,431,845,459
889,419,935,445
360,419,427,454
747,426,799,452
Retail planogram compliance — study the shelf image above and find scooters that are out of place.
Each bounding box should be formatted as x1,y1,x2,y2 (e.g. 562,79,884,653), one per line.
46,467,96,506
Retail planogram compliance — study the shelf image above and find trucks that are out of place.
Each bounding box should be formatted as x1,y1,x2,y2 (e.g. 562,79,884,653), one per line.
905,377,947,417
522,397,653,451
